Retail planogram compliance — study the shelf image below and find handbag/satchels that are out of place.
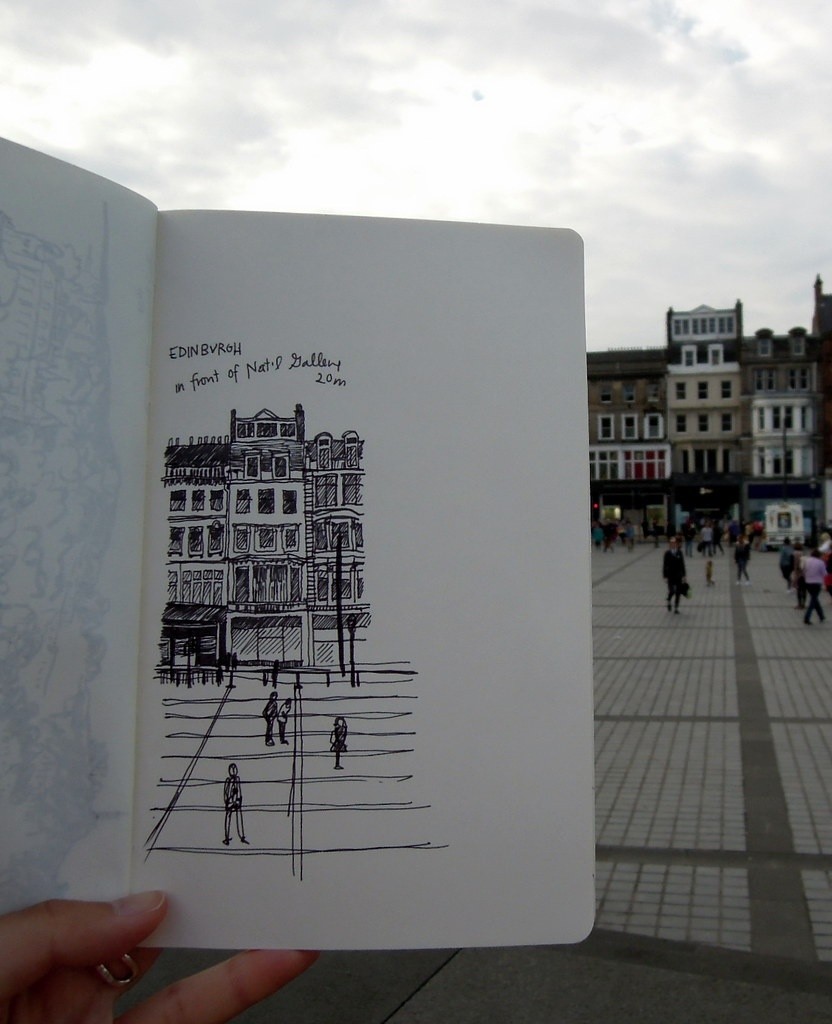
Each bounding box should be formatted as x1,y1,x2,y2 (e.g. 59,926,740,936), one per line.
681,581,690,597
789,554,794,569
697,542,704,552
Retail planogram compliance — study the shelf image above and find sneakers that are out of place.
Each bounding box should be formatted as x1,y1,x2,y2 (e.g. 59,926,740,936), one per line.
735,581,740,587
745,581,751,586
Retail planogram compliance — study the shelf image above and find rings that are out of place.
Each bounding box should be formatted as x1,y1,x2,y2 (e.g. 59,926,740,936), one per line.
94,952,139,990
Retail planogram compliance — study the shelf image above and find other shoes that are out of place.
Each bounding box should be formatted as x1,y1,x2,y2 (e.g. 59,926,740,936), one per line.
667,604,672,611
786,589,794,593
820,617,826,623
675,610,681,614
803,620,812,625
794,605,807,610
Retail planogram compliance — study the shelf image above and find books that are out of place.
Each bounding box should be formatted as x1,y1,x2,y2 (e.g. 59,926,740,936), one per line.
0,131,598,951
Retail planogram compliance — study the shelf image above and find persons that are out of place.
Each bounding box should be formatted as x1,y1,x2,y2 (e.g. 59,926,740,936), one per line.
0,888,316,1022
804,550,827,625
819,532,831,598
663,536,686,614
701,522,714,557
593,515,769,553
793,542,806,611
735,535,751,585
706,560,715,587
779,536,795,593
